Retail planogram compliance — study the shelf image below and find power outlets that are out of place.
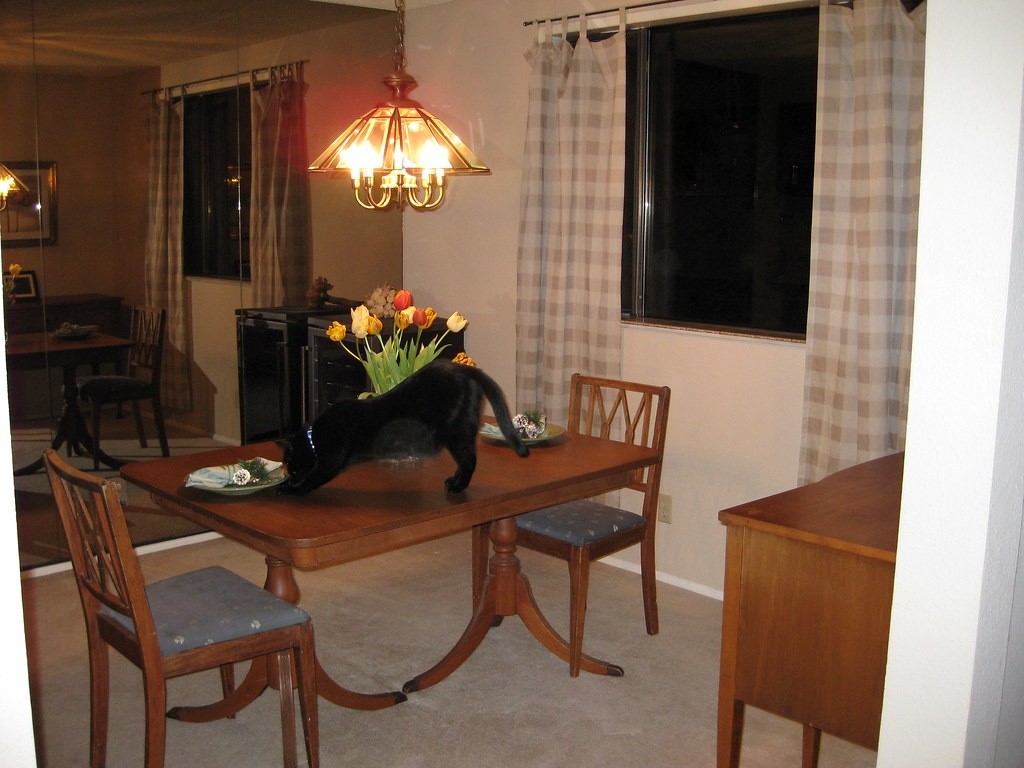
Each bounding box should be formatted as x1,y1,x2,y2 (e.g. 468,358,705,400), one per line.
658,493,672,524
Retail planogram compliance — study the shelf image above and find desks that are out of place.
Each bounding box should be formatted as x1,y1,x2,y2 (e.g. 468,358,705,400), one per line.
3,293,125,419
717,450,906,768
5,329,138,477
117,415,664,724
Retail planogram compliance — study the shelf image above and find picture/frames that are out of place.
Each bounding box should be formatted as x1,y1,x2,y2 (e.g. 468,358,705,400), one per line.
3,271,40,303
0,160,58,249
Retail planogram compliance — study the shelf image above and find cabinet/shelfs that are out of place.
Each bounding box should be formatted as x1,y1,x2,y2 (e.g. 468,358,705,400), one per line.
235,303,360,446
300,313,463,426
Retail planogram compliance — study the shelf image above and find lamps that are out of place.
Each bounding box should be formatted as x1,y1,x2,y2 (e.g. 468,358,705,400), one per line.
305,0,490,210
0,163,30,212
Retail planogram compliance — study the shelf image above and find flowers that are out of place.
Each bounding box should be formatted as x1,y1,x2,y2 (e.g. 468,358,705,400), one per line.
2,263,23,328
325,279,476,400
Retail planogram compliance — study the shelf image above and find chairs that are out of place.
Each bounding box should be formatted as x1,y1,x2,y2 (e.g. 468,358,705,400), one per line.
472,373,671,678
66,304,171,470
42,447,320,768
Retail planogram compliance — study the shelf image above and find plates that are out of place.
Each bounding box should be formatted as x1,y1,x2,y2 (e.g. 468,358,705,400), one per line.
479,421,566,447
183,463,289,497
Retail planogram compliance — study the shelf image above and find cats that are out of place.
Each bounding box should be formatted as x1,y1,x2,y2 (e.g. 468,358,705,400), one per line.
273,358,531,498
